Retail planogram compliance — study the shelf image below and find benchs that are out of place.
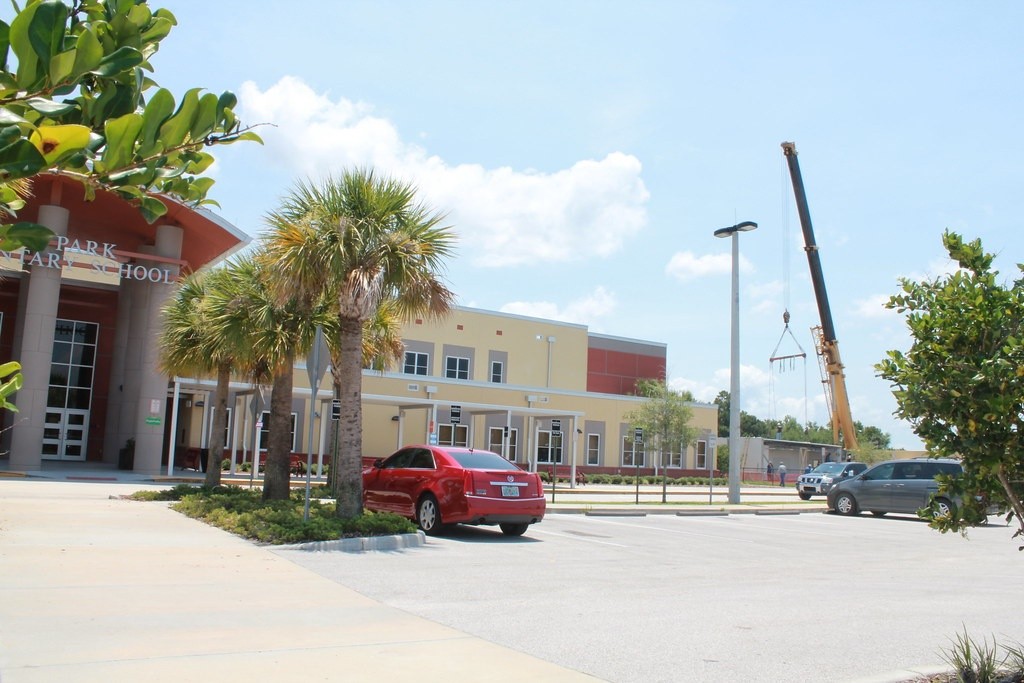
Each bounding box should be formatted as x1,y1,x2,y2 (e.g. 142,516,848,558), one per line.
259,454,303,478
548,466,585,486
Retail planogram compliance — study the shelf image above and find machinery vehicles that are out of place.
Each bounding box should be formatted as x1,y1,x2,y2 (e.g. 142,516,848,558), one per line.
779,139,963,467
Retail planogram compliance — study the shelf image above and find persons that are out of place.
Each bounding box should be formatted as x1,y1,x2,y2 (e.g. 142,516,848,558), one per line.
805,463,814,473
825,452,831,462
846,453,851,462
767,461,773,481
778,461,787,487
776,422,783,439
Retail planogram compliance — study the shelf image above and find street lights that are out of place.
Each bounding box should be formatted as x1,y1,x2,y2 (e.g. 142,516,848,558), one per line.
714,220,757,505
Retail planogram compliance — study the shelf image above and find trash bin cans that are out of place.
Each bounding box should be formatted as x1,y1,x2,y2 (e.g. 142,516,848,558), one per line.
200,449,209,473
119,447,134,470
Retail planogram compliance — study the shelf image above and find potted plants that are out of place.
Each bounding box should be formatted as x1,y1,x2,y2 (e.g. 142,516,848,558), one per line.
119,437,136,470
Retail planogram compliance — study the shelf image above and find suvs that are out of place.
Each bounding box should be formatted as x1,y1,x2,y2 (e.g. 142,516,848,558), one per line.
826,457,976,525
795,462,873,500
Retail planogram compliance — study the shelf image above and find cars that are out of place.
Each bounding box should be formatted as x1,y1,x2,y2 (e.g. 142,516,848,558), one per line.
361,445,546,538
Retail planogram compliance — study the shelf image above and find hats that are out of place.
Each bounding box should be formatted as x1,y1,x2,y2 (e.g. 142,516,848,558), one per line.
780,462,783,464
777,423,780,425
808,464,812,467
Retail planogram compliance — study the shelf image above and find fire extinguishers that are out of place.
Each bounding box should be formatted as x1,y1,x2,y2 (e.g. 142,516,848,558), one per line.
430,421,433,433
